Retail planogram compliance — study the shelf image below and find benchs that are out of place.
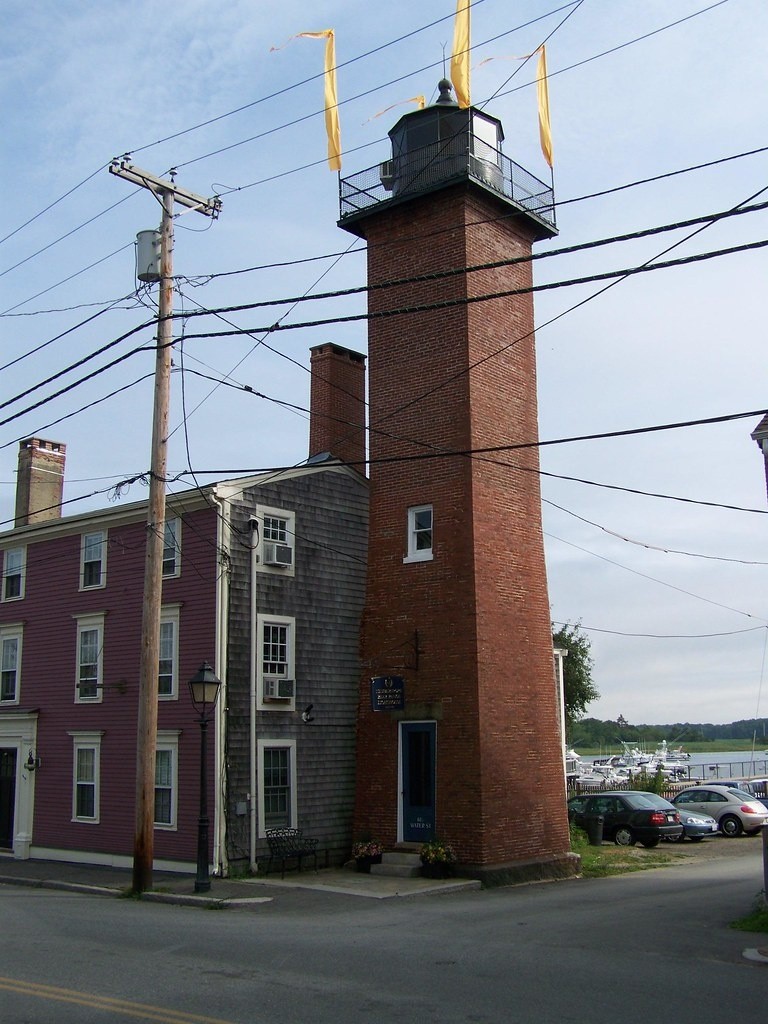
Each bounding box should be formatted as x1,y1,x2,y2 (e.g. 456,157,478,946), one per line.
266,827,319,881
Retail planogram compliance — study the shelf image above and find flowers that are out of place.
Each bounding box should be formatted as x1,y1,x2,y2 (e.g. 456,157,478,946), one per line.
416,839,457,865
350,832,386,860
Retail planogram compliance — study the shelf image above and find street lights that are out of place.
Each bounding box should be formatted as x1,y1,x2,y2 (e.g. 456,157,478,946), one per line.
187,659,212,893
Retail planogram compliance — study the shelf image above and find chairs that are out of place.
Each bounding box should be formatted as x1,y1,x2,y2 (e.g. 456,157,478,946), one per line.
592,800,600,813
717,795,723,800
700,793,706,801
603,802,613,814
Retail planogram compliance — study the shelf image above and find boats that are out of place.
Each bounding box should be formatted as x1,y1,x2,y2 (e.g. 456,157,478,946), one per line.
565,732,731,787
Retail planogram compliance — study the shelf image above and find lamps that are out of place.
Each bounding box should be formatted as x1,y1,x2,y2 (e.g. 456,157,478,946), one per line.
23,747,43,772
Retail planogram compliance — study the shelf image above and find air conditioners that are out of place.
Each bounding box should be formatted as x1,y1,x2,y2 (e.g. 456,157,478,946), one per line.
264,678,295,699
380,161,395,191
263,544,293,566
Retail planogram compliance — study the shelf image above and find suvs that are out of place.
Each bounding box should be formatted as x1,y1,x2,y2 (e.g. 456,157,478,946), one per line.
566,790,684,849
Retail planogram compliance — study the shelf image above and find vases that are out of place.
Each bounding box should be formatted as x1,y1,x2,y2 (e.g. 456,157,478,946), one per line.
421,859,449,880
351,854,382,874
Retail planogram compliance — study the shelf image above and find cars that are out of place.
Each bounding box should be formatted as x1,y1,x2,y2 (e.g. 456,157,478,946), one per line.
665,784,768,838
674,806,722,842
703,780,755,802
